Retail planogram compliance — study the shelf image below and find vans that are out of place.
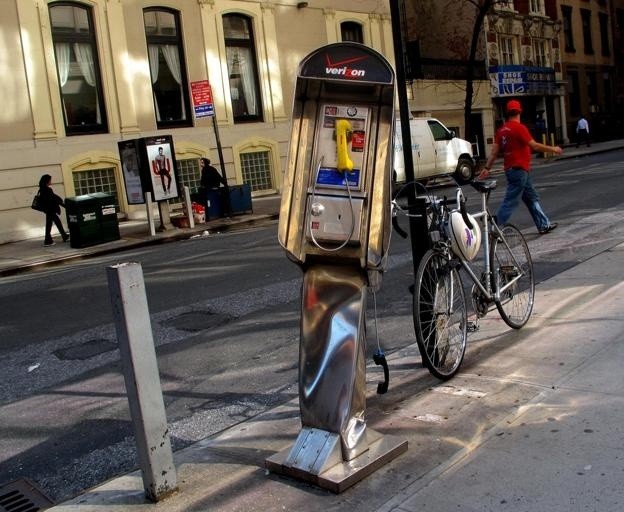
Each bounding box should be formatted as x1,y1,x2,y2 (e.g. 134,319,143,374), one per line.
393,115,475,192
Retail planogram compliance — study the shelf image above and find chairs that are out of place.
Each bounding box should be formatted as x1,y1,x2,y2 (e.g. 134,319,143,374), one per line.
190,185,254,222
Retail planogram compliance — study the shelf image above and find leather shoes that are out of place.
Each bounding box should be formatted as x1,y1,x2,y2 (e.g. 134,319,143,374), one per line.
540,223,558,233
488,230,503,243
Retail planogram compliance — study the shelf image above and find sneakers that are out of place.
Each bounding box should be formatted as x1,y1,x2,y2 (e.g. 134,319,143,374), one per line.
44,242,56,247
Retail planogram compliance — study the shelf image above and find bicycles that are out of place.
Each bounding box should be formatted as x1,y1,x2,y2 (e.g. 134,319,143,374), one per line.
393,169,535,381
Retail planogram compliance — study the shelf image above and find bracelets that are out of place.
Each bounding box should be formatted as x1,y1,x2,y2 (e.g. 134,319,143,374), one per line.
484,167,490,171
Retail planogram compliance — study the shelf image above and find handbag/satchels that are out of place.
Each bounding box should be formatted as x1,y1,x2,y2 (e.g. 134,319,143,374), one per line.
32,196,42,210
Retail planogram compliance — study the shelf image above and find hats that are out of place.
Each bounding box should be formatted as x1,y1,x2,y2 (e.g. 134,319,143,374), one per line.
505,99,522,114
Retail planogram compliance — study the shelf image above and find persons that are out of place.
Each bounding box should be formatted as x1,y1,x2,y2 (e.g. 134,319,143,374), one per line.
480,100,563,242
198,158,228,198
155,147,171,195
575,113,592,148
39,174,70,246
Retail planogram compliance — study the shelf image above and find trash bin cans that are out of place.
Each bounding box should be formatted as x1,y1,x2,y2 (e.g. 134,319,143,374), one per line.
190,184,254,222
65,192,121,249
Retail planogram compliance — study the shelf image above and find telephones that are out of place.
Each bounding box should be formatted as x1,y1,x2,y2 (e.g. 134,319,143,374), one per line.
335,120,353,173
373,353,389,394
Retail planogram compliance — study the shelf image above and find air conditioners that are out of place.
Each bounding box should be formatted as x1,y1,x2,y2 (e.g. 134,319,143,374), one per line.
487,42,499,60
521,45,533,61
551,48,560,63
